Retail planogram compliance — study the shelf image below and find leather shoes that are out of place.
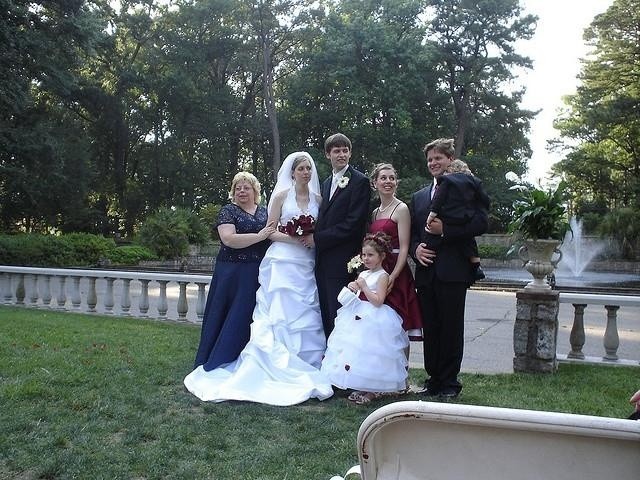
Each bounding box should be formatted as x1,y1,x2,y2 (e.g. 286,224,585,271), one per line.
416,385,457,397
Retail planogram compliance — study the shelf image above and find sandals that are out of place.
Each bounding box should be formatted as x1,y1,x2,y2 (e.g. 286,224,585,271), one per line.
348,391,380,405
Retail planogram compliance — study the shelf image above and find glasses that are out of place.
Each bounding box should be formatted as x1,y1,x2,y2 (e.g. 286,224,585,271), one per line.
234,187,252,191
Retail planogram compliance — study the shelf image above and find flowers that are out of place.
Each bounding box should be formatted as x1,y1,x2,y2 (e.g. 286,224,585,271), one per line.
279,215,318,239
346,254,364,279
336,176,351,189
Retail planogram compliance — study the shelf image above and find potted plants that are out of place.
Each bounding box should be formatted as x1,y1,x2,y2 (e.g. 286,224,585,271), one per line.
503,171,579,296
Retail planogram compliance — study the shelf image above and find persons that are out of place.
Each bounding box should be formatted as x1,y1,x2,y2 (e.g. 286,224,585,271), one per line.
418,158,490,280
370,162,424,372
193,172,276,373
409,139,489,398
299,132,371,349
183,151,335,407
627,391,640,420
319,232,409,405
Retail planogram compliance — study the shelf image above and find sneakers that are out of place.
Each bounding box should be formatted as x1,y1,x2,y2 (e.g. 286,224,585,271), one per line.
471,265,485,279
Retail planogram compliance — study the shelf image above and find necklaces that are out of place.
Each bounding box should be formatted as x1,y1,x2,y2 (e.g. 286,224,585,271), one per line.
379,197,396,213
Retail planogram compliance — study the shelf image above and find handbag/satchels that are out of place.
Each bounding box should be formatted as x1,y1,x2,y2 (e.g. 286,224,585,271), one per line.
337,286,362,315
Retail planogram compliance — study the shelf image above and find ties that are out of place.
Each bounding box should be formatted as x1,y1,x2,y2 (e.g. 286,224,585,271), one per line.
329,178,336,201
